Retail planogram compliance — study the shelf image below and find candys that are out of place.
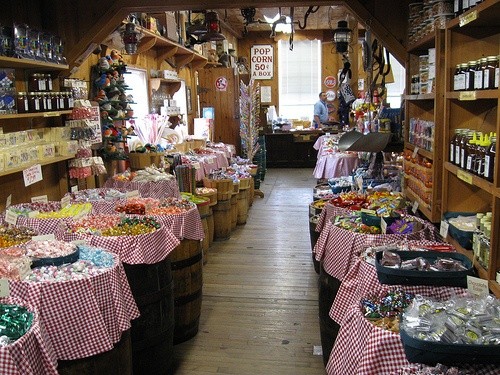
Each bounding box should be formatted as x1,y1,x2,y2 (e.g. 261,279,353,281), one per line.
0,188,193,347
312,175,500,375
180,142,253,204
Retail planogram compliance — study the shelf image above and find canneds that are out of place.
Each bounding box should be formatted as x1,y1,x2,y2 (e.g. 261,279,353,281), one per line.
448,128,496,184
17,74,75,113
453,55,500,92
408,0,456,95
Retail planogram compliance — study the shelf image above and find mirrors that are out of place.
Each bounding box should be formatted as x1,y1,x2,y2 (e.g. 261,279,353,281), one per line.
159,77,188,140
120,65,151,152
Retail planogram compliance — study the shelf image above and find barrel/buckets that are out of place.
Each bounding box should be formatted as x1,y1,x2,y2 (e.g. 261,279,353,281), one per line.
229,176,254,232
308,181,332,273
54,324,139,375
318,257,341,368
119,233,176,375
203,179,233,242
193,187,217,265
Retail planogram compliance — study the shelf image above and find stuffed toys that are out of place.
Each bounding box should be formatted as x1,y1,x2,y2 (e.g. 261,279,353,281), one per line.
96,50,134,152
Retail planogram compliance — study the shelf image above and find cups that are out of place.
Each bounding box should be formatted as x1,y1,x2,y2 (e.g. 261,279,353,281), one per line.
171,232,204,345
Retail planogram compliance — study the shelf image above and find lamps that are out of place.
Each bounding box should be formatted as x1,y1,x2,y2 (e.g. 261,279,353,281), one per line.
333,5,353,54
261,8,295,33
200,9,226,41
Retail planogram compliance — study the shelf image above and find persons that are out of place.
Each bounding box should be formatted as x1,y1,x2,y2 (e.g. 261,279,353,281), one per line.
313,92,328,128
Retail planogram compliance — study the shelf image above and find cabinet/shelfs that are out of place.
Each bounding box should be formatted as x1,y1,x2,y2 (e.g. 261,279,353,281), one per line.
403,0,500,300
0,56,72,119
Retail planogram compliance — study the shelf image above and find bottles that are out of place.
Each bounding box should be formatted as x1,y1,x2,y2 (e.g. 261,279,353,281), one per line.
410,74,420,94
473,212,492,272
449,128,497,183
454,54,500,91
